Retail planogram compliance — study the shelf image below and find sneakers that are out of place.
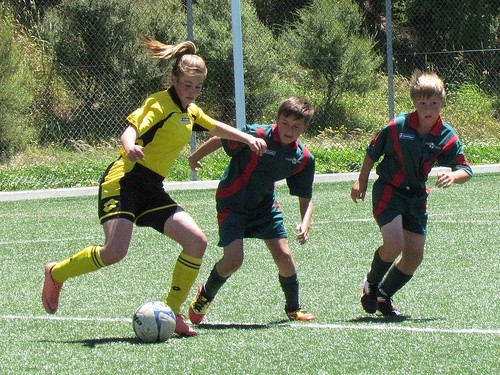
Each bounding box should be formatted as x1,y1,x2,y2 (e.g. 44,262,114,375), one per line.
41,263,64,315
285,310,315,321
360,278,381,313
376,291,403,317
189,283,210,325
174,314,197,336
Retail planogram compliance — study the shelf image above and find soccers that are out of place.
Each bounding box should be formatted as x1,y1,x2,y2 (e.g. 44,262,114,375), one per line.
132,301,176,342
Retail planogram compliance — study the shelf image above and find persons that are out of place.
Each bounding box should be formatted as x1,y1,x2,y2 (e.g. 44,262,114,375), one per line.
188,97,316,325
42,36,267,337
351,73,472,324
